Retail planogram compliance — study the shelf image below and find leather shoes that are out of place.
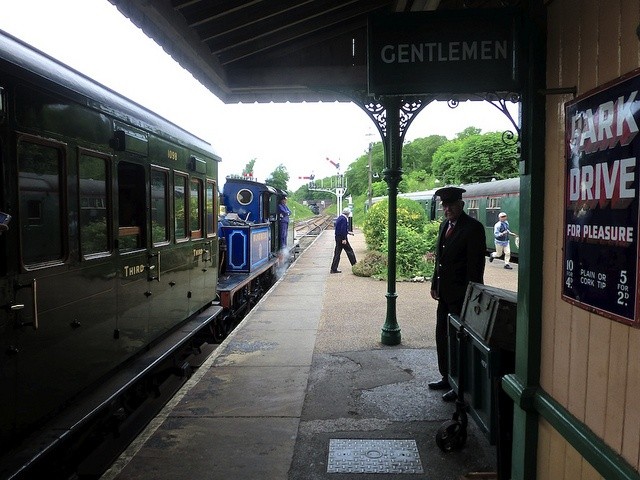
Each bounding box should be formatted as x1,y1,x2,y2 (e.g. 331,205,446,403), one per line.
428,379,450,389
504,264,512,270
490,254,493,262
442,389,457,402
330,269,341,273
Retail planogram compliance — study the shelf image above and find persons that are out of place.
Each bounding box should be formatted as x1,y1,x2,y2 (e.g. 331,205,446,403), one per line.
278,196,292,251
489,211,517,270
330,207,357,273
428,187,487,402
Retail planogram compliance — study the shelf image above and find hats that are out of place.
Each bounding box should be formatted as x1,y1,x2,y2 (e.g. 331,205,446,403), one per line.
435,188,466,199
499,212,506,218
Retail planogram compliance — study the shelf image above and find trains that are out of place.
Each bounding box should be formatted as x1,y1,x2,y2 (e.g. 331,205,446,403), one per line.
1,28,288,476
364,177,521,263
